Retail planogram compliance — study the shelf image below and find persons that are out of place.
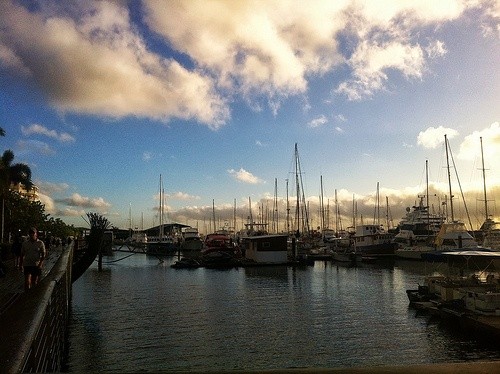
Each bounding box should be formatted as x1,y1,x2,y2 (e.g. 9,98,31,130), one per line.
41,233,73,256
18,227,46,298
11,237,25,273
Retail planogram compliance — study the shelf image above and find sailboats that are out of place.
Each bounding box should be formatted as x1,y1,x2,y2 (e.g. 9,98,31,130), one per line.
146,173,184,256
204,133,500,260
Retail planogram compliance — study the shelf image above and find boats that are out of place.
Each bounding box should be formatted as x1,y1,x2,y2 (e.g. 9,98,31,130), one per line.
407,248,500,344
180,226,203,250
